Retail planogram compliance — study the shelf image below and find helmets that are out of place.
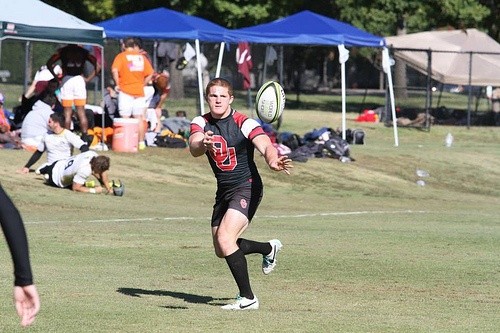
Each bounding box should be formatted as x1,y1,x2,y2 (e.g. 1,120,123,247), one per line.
113,180,123,196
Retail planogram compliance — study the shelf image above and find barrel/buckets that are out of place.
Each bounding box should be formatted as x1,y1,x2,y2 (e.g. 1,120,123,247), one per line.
113,118,139,152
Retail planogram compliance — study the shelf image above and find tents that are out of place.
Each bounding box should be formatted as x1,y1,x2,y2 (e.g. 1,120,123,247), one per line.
215,11,399,147
0,0,106,139
84,8,227,125
383,27,500,130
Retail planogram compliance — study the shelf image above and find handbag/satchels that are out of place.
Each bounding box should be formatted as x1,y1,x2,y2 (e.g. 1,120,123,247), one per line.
345,128,365,145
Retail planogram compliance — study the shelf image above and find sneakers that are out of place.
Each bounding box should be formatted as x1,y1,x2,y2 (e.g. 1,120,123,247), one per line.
221,296,259,310
262,240,281,274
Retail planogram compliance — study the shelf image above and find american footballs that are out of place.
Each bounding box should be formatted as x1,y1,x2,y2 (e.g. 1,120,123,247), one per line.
255,81,286,124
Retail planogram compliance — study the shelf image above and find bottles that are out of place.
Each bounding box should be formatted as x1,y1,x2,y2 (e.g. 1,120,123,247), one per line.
446,132,453,148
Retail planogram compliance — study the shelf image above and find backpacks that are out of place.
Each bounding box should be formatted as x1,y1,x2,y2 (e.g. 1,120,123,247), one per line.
326,135,348,157
305,128,331,145
155,136,187,147
281,133,301,149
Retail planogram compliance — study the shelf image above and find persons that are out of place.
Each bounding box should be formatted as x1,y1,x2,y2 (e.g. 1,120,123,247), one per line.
36,150,113,197
20,92,92,150
127,37,154,135
188,77,293,311
23,112,89,174
1,185,42,328
0,93,364,164
111,36,146,149
46,38,96,133
